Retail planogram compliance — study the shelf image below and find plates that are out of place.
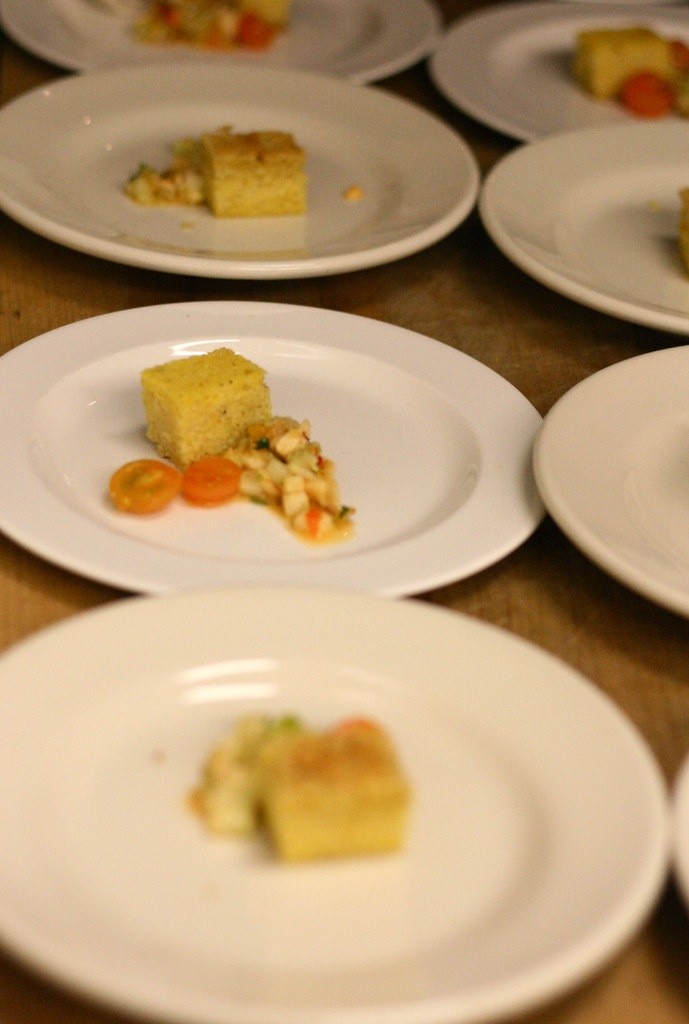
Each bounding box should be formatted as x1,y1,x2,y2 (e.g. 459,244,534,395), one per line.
1,65,479,280
1,1,436,83
672,750,689,909
478,121,688,340
0,589,672,1024
532,346,688,620
0,303,547,595
426,0,689,140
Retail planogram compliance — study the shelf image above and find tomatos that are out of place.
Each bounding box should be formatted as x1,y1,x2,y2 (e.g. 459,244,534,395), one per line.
110,456,241,516
237,16,271,45
621,72,673,113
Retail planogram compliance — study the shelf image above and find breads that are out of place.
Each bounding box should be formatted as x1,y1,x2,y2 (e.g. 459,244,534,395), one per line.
141,347,273,464
201,132,308,216
263,713,411,859
574,29,673,97
